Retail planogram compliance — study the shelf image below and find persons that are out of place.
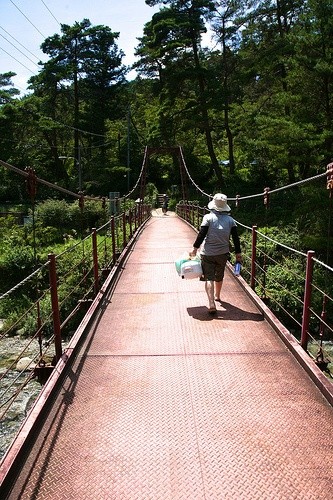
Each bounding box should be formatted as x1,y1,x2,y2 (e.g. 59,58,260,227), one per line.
189,194,244,314
161,194,169,215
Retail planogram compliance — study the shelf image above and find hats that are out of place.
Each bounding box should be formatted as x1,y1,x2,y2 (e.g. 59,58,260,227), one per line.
207,193,231,212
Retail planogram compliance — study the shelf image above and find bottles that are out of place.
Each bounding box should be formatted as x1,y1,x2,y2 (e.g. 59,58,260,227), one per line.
234,261,241,275
